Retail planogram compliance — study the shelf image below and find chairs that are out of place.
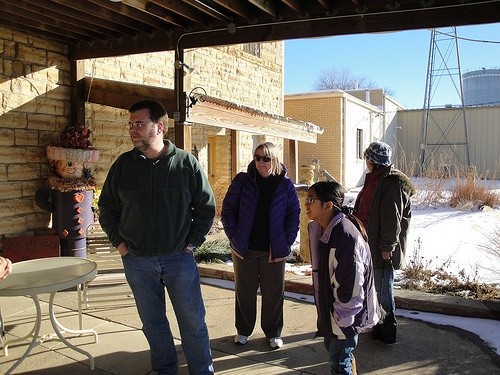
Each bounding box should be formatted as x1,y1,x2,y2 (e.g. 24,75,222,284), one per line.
77,224,137,330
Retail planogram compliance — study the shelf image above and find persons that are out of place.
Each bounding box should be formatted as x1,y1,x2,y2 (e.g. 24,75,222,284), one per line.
304,181,386,375
98,100,217,375
221,142,301,349
353,141,416,346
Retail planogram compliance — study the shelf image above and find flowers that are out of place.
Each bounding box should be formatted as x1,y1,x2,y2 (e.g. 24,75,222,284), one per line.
58,123,92,148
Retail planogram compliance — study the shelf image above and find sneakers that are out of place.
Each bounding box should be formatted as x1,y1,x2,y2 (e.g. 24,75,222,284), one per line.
269,337,283,349
235,335,248,344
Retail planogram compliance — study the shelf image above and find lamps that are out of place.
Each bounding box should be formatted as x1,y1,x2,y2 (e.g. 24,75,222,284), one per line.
182,63,196,74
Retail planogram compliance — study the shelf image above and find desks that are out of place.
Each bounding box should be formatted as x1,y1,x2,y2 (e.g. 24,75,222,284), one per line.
0,256,99,375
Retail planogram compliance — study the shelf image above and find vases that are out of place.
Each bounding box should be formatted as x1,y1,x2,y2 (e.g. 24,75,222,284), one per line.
46,146,99,180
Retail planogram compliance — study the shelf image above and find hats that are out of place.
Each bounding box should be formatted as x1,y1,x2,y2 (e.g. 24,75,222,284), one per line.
364,142,392,166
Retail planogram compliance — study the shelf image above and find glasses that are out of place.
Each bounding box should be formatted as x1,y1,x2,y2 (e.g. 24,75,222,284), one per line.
125,121,158,129
253,154,273,162
305,197,319,204
